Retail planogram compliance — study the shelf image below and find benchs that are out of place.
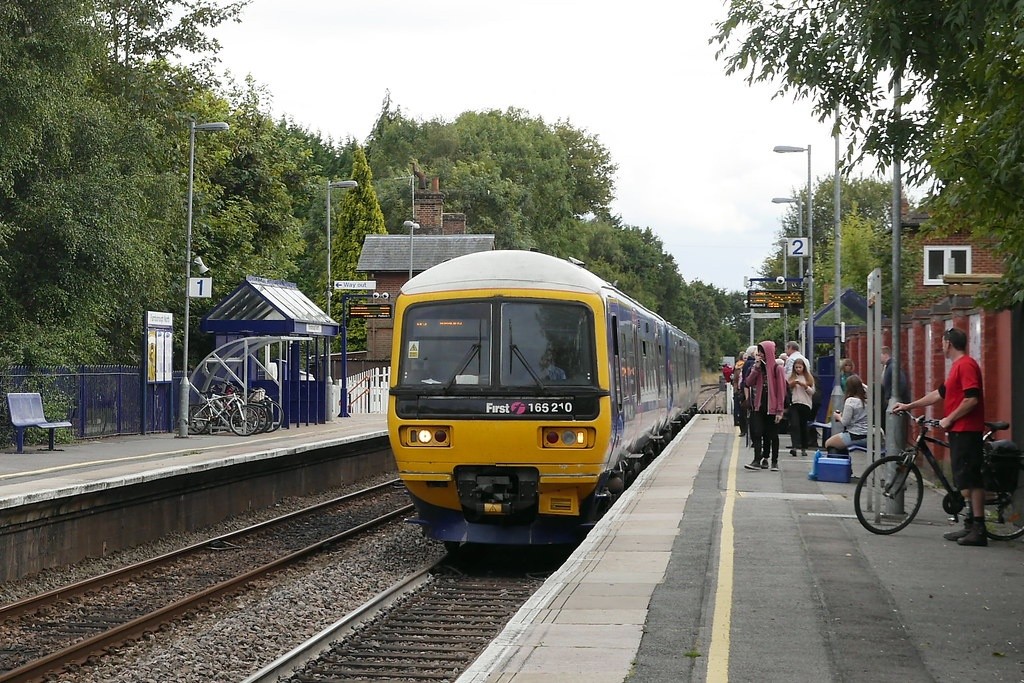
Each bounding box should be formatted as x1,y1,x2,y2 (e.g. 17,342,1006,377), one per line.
4,393,72,455
813,421,885,477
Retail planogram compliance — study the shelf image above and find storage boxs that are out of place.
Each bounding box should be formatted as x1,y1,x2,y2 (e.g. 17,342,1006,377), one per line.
818,456,850,482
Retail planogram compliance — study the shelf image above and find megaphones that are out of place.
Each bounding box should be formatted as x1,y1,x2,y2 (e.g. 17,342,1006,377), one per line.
195,257,209,274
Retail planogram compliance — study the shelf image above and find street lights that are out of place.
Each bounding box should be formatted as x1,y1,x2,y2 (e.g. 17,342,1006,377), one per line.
770,145,813,377
178,121,229,439
404,177,419,279
326,180,358,422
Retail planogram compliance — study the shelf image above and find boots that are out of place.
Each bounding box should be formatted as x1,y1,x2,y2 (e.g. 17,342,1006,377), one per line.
944,517,972,540
958,521,987,546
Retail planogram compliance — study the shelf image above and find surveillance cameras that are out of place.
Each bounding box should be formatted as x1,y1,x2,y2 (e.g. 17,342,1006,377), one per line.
373,292,379,299
383,292,389,299
777,276,784,285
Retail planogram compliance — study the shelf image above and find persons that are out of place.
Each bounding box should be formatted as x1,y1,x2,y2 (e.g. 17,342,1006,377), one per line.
733,340,815,472
825,358,867,474
522,338,565,380
881,346,906,434
891,329,988,546
722,362,733,382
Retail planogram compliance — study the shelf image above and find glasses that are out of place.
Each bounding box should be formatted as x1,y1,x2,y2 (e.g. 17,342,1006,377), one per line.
947,327,954,338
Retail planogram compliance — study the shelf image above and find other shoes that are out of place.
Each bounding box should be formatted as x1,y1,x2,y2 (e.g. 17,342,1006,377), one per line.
770,459,779,471
739,431,746,436
745,459,760,470
801,450,807,457
761,459,768,468
790,450,796,455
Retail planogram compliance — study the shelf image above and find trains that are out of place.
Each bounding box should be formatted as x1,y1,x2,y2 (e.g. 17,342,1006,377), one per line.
388,247,702,555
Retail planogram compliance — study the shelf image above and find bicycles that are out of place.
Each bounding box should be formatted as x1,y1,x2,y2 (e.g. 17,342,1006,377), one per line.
187,382,284,436
854,406,1024,541
